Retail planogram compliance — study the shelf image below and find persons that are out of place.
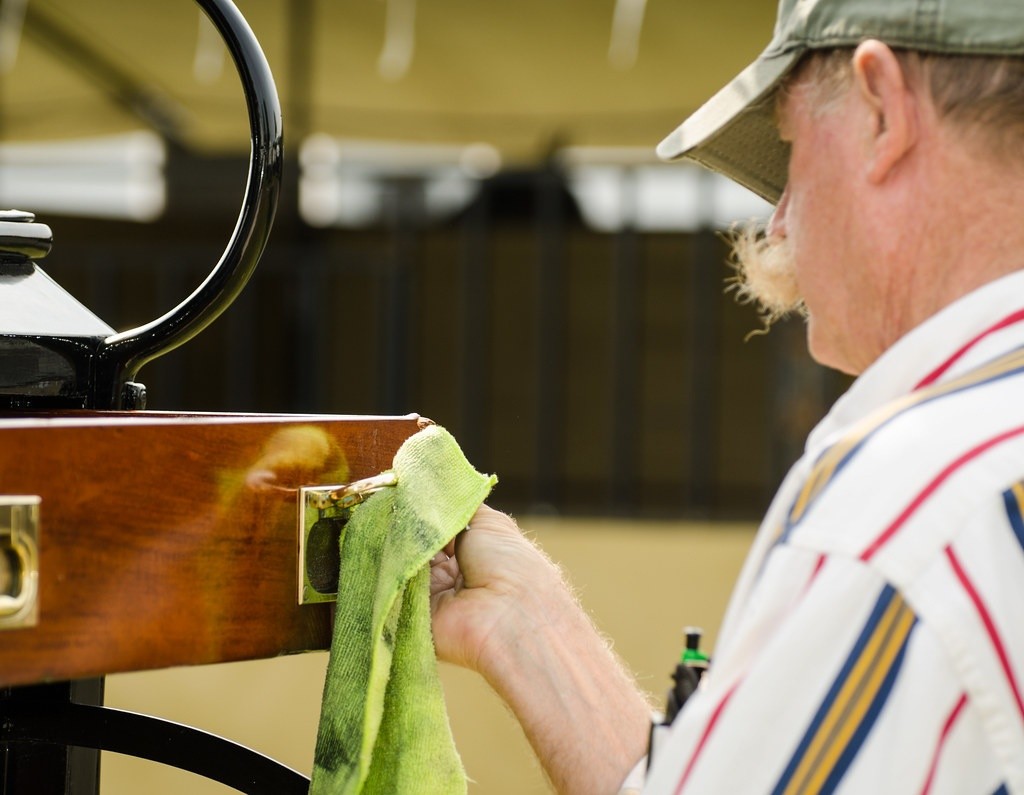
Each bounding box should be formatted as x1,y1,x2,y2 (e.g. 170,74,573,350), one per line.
428,0,1024,795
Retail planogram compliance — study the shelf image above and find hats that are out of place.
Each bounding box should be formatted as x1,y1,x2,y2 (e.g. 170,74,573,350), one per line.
657,0,1023,207
